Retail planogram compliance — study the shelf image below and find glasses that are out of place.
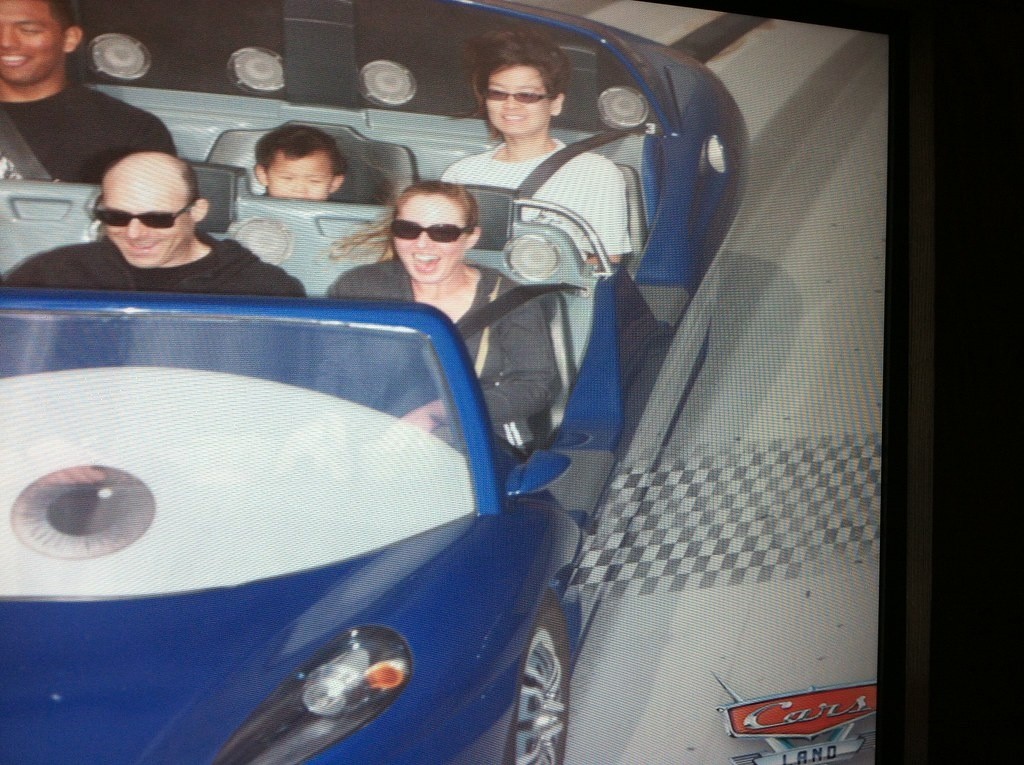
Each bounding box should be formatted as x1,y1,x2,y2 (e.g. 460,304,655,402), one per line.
93,195,196,228
390,220,473,243
485,89,553,104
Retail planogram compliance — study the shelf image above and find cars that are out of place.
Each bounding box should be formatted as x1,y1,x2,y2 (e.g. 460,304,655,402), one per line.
0,0,747,765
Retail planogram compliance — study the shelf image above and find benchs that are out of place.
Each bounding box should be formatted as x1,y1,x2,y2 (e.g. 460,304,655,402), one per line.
0,83,645,427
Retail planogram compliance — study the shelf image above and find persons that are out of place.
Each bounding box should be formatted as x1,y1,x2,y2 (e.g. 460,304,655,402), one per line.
254,125,346,200
324,180,562,457
0,0,177,185
444,28,632,263
0,150,306,382
